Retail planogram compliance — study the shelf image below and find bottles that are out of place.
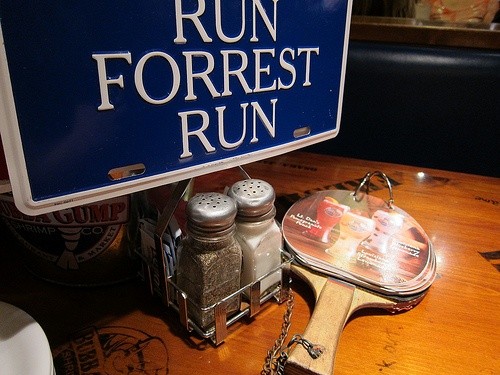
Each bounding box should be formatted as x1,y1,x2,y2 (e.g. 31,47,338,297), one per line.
175,192,242,334
226,179,283,303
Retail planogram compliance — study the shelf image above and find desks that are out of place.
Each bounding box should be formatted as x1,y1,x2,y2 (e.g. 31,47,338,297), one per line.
0,151,500,375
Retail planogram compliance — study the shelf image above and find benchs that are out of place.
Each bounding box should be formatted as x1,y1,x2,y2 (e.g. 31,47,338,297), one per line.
296,15,500,177
